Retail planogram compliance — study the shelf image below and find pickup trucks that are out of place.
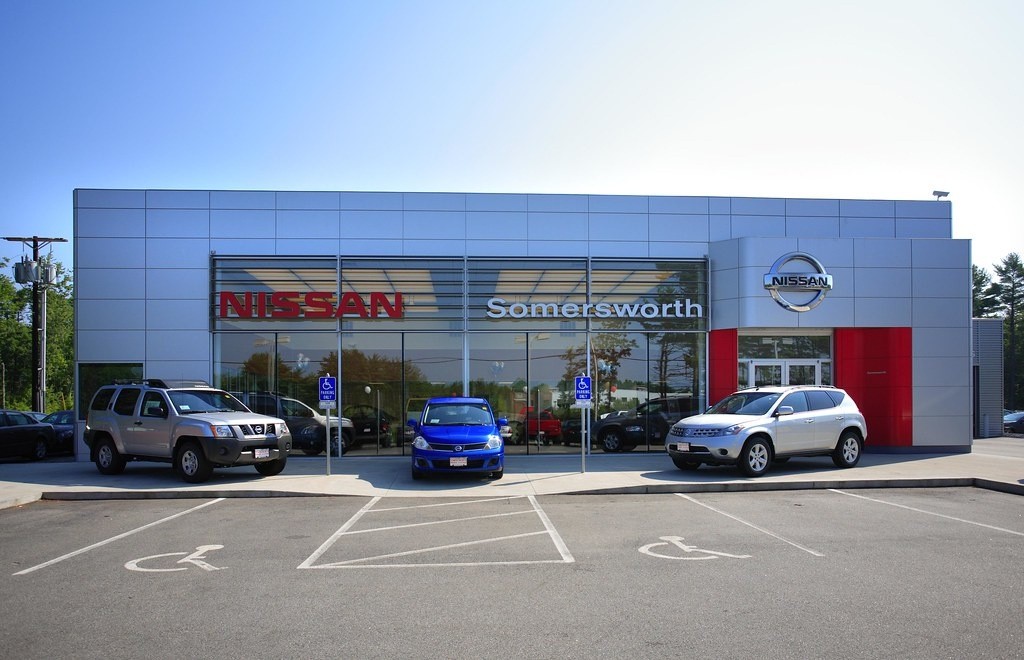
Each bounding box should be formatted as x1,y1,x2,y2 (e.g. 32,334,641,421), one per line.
517,410,562,445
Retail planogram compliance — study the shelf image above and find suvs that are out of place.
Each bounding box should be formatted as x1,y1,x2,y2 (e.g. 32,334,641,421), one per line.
596,393,701,454
84,378,293,484
406,397,428,425
664,382,868,478
563,420,593,447
224,391,354,457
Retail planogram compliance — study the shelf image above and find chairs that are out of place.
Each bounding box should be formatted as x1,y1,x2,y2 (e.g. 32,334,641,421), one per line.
467,406,486,421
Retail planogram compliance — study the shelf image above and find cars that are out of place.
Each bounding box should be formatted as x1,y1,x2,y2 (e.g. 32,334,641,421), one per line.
1004,411,1024,432
497,424,513,444
408,397,508,480
338,404,414,450
0,410,54,462
40,410,74,456
20,412,56,422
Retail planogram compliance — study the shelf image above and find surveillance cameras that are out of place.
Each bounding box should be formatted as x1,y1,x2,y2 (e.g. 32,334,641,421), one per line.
933,191,950,197
